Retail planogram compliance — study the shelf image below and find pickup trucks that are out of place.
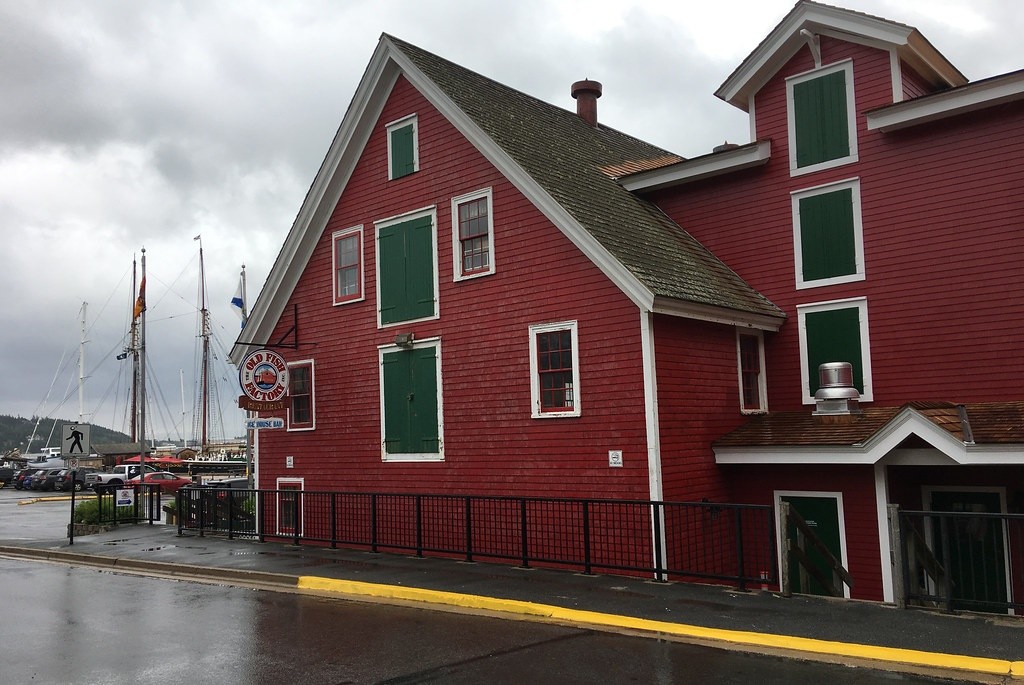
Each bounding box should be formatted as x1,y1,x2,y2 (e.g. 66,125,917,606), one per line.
84,464,157,495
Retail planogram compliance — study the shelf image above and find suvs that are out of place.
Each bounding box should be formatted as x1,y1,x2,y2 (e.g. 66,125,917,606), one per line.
11,468,105,492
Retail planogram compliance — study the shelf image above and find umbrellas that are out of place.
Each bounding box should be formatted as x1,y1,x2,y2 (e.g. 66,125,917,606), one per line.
123,455,154,463
154,455,183,472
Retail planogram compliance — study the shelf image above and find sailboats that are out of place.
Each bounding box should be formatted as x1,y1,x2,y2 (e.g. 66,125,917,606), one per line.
14,233,255,475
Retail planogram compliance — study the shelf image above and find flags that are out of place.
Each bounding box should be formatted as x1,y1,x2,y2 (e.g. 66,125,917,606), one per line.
230,276,247,328
134,277,146,318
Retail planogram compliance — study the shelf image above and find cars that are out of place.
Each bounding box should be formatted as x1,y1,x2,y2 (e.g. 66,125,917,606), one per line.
123,471,193,495
206,478,249,509
